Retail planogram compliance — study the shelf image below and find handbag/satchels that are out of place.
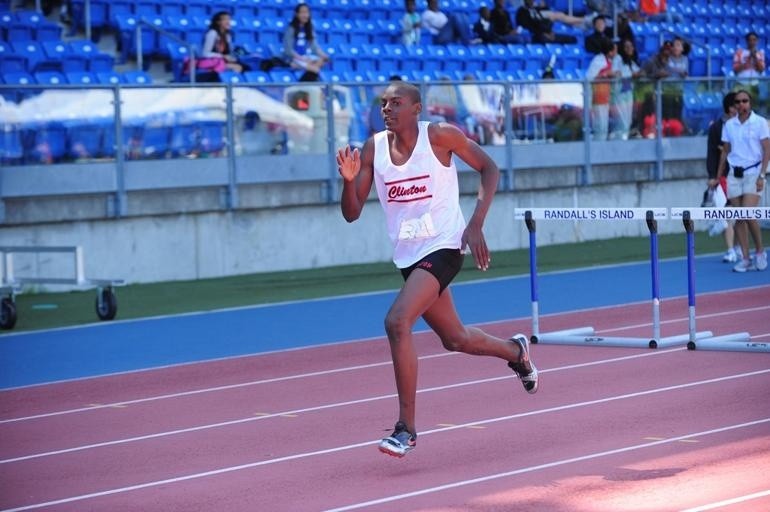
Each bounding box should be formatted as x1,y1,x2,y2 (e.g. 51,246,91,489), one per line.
734,167,743,177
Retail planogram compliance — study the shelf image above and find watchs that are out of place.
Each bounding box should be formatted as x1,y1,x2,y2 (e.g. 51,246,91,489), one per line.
758,172,767,179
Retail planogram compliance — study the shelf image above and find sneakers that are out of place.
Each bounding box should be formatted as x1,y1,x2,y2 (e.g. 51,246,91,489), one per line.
723,245,767,272
378,421,416,458
508,333,539,394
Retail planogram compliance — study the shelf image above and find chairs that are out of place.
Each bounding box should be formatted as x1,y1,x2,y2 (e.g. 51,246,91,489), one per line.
1,0,770,167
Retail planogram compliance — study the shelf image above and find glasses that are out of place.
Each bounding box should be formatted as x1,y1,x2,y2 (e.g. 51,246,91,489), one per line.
734,99,749,104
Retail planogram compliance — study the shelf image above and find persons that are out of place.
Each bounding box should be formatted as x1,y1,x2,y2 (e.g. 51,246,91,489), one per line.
201,11,245,76
585,15,610,53
634,36,689,137
532,1,597,28
428,74,506,145
586,38,642,141
730,30,766,110
401,0,422,47
707,90,745,260
421,0,449,45
336,81,538,457
709,90,769,273
617,11,634,40
474,6,497,46
538,71,582,141
490,0,530,44
283,3,332,75
516,0,555,44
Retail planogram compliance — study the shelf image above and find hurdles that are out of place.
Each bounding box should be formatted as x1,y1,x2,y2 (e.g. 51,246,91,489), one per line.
512,207,712,349
670,206,770,352
